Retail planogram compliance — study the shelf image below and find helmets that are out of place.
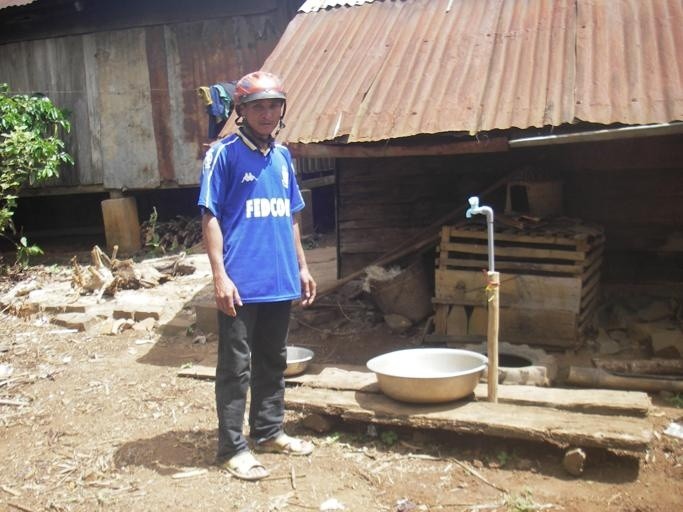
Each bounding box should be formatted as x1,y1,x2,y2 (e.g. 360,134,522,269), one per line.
232,71,288,106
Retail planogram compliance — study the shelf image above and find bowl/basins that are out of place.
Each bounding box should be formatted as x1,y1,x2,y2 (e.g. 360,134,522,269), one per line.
365,347,488,404
283,346,314,376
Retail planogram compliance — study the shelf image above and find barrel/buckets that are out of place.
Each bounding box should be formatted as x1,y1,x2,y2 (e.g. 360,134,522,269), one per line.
372,255,434,324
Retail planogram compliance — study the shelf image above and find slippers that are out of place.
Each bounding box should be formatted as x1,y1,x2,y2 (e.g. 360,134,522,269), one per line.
255,431,315,457
221,449,270,481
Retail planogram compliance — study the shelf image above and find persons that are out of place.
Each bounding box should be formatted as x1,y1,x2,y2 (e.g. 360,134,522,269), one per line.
198,70,318,482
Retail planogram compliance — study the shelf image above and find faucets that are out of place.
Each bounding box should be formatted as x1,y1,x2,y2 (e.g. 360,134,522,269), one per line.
466,197,483,218
